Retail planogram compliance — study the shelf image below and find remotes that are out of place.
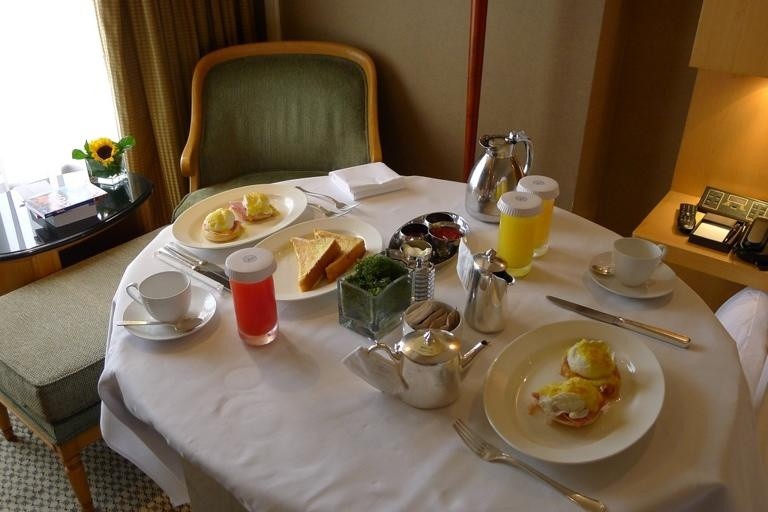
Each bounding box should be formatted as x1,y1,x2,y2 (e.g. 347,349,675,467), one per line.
679,203,696,232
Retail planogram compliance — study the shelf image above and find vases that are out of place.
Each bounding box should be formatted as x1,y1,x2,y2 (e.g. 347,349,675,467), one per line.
86,151,129,190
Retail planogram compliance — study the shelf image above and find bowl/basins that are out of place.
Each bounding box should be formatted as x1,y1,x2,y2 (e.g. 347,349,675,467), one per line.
396,213,465,262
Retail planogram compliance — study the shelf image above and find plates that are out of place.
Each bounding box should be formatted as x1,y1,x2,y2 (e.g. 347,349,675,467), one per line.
385,211,471,267
172,183,308,249
481,319,667,464
250,216,383,302
587,249,676,300
121,286,216,341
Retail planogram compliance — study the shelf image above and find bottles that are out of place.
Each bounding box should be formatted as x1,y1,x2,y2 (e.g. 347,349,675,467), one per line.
515,174,561,262
463,248,516,336
495,192,543,280
408,257,438,302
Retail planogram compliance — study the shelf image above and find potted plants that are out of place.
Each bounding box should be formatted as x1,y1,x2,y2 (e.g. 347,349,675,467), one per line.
337,255,416,343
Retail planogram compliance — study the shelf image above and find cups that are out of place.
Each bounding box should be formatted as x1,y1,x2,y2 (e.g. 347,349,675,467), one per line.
610,236,668,288
222,248,280,351
124,270,192,326
401,302,464,341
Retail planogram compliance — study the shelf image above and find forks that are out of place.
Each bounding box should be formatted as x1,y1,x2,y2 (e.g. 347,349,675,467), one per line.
450,414,608,511
295,186,359,218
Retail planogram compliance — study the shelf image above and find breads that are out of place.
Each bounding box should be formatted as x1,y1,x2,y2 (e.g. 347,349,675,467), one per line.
291,237,341,291
202,208,240,242
529,339,621,427
229,192,279,224
312,229,367,283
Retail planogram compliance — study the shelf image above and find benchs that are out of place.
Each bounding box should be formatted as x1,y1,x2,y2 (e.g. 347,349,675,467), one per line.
0,224,171,512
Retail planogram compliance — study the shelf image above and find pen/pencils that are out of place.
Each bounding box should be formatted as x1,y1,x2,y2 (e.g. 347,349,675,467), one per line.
724,223,741,243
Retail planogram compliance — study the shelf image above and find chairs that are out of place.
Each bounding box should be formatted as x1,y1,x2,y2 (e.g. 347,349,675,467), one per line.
172,41,382,223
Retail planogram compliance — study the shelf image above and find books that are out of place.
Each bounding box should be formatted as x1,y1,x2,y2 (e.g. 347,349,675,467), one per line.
25,179,109,228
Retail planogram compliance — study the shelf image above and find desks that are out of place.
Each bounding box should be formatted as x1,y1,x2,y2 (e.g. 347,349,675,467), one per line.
631,190,768,293
0,171,153,296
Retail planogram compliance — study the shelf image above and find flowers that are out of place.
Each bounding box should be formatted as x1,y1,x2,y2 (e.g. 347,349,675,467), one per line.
72,135,135,177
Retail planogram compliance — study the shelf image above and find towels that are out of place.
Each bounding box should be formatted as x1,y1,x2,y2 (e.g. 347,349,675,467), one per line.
328,162,406,200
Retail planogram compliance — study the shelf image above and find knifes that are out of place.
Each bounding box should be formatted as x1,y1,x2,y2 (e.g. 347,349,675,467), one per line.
544,293,691,350
154,241,230,294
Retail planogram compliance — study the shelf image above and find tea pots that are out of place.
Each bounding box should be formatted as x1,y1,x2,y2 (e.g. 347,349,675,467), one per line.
463,124,535,225
342,329,489,413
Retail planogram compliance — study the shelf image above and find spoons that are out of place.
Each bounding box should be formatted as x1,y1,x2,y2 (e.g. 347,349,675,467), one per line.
590,261,614,277
115,317,202,335
